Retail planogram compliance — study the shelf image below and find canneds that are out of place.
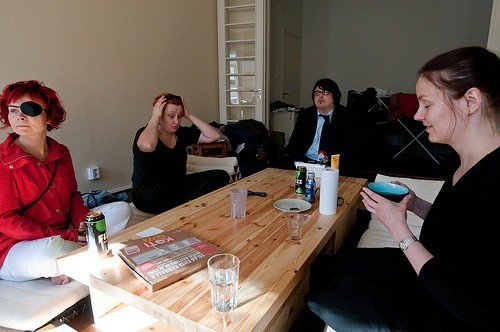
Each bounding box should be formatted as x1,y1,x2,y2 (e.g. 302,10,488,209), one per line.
295,166,307,194
84,210,108,257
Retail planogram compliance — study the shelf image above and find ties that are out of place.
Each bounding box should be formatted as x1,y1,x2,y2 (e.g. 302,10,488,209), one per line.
318,114,331,152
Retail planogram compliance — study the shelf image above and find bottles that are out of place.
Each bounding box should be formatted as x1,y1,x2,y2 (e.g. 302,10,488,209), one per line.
305,172,317,203
273,199,311,212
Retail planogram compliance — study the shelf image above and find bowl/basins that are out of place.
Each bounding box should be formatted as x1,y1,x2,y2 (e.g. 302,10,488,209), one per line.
367,182,409,203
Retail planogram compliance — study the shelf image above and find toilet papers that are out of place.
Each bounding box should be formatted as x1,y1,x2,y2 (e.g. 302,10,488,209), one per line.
319,168,339,215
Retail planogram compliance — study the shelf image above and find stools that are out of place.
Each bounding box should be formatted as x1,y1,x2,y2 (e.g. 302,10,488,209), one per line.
189,142,227,159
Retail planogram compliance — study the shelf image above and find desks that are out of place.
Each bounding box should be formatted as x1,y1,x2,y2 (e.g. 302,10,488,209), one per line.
53,166,371,332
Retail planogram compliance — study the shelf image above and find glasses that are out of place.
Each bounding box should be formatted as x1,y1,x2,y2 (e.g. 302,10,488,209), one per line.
313,90,331,96
165,95,182,101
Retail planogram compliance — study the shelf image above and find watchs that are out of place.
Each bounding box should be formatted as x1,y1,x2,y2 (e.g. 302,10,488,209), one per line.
399,236,418,251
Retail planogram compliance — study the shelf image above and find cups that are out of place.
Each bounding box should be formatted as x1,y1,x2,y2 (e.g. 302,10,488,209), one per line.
286,214,303,241
208,254,240,312
230,189,247,218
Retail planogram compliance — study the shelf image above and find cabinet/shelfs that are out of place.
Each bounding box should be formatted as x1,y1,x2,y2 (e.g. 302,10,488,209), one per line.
271,111,301,147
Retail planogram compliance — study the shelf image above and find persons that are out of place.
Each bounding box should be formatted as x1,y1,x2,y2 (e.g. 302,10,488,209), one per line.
131,93,230,215
307,45,500,332
0,80,134,285
284,78,361,178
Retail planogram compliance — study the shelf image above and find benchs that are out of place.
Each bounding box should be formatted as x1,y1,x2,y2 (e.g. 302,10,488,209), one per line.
0,155,241,332
